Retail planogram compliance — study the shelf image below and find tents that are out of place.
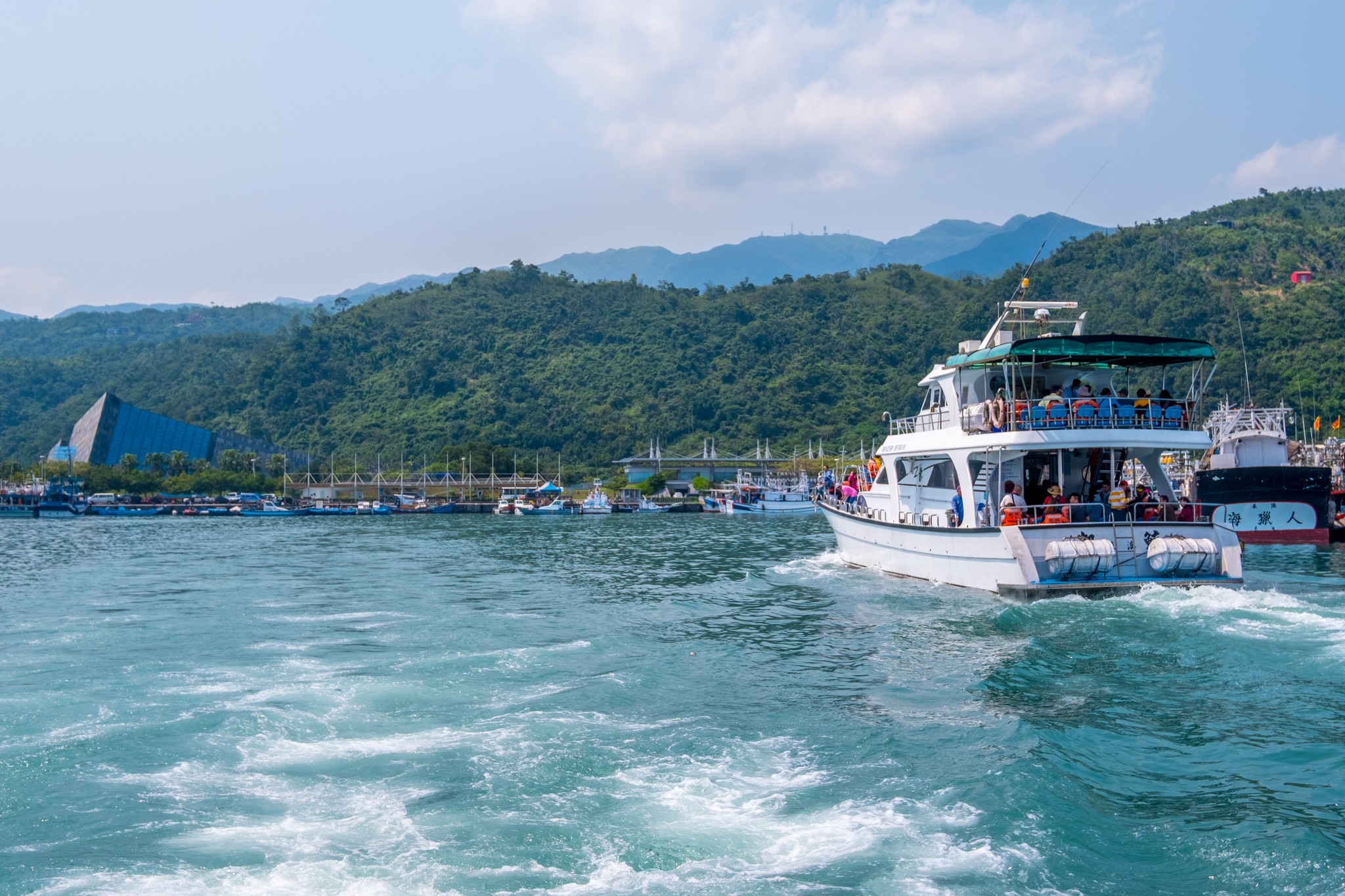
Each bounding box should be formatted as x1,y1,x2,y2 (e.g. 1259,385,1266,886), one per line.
534,480,561,505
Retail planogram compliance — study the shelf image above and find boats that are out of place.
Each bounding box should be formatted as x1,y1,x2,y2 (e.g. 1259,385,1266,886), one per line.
816,158,1246,605
0,366,1345,546
1185,317,1333,547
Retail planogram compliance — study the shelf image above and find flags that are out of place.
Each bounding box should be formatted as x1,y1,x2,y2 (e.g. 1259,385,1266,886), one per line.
1332,415,1340,430
1313,416,1320,431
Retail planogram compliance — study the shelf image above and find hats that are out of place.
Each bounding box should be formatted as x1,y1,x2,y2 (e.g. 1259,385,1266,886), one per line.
1075,386,1092,396
1102,387,1111,395
1047,485,1061,495
1136,484,1145,492
1117,389,1129,395
1120,480,1129,488
1145,388,1152,395
1053,384,1063,392
957,486,961,491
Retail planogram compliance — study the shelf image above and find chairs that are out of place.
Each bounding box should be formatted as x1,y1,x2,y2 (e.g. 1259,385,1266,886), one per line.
1015,405,1184,430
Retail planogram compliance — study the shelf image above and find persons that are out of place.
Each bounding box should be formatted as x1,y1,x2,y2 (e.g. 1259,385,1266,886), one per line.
1003,378,1196,429
866,455,880,485
830,474,842,500
812,465,832,501
848,471,861,491
835,483,858,513
1004,479,1198,522
951,486,964,528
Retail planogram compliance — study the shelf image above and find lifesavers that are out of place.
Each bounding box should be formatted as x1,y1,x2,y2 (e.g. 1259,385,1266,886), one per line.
991,396,1005,429
981,399,992,430
